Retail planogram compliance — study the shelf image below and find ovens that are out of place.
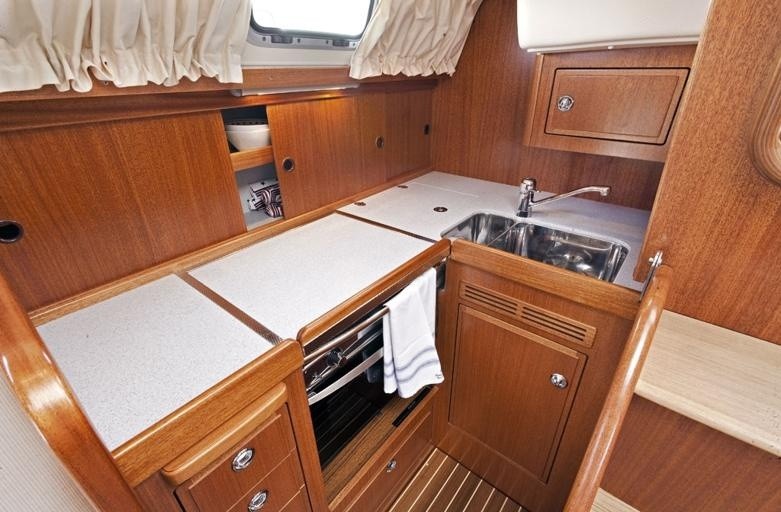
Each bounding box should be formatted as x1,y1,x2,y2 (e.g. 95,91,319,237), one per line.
302,264,452,475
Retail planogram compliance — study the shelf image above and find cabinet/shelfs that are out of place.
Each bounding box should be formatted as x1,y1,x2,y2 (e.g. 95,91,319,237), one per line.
0,170,699,512
521,43,697,166
0,66,435,329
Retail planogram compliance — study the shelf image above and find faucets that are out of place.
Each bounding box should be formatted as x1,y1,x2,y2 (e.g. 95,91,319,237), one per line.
518,177,612,217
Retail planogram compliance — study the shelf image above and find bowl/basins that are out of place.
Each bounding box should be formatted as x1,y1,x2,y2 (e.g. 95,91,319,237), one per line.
225,121,270,151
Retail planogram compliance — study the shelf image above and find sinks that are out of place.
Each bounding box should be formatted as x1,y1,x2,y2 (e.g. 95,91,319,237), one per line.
487,222,633,284
439,209,518,247
